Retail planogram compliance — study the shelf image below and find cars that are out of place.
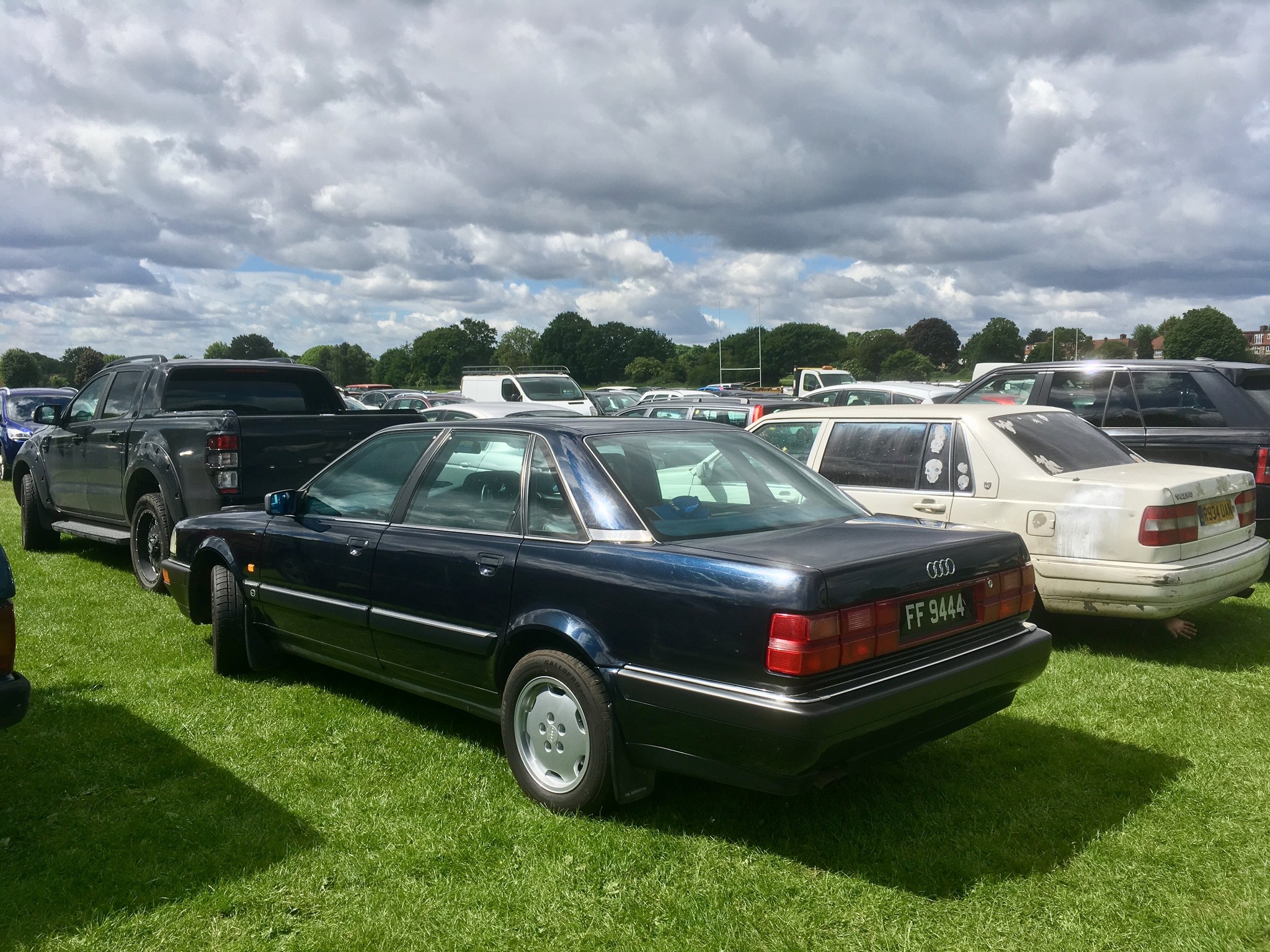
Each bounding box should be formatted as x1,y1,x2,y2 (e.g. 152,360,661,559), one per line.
347,365,968,427
656,402,1268,623
162,410,1055,814
945,360,1269,527
1,383,79,480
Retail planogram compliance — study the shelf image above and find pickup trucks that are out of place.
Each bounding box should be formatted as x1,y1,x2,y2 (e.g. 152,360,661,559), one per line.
8,351,432,591
